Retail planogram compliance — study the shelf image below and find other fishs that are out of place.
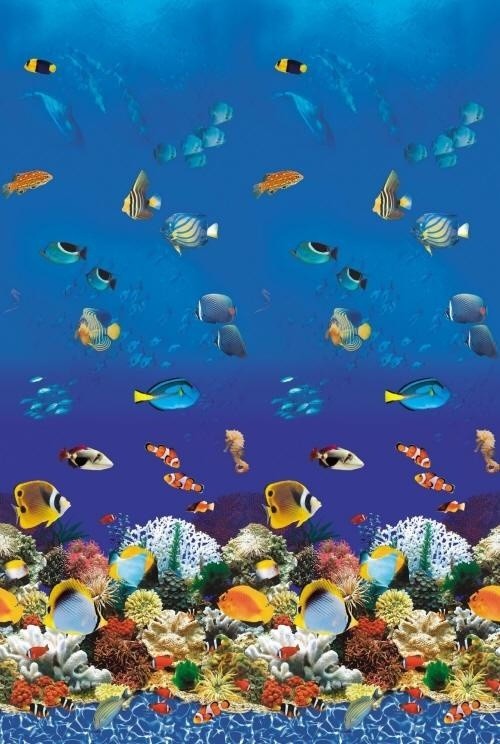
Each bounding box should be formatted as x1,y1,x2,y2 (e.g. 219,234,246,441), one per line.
0,49,500,744
